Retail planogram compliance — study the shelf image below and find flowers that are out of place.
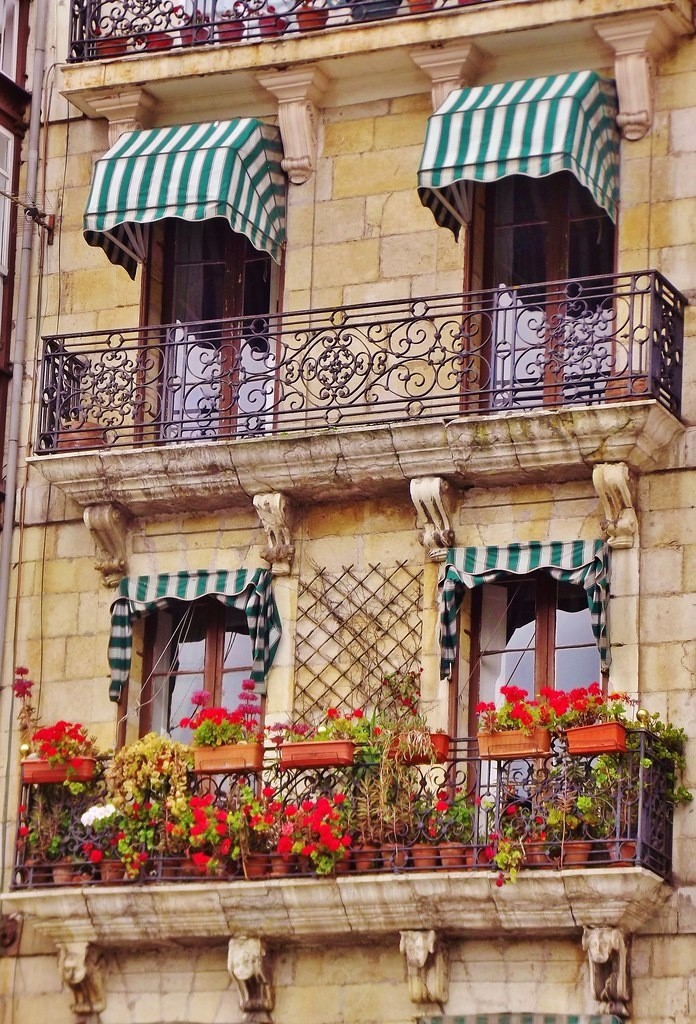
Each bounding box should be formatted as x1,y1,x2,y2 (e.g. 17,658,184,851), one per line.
29,718,98,767
87,0,319,38
470,684,629,731
17,780,643,882
272,708,370,745
378,667,432,732
182,678,265,745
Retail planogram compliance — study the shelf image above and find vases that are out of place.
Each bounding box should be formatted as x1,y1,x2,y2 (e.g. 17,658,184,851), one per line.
476,728,551,757
606,837,634,867
179,25,209,46
330,848,350,874
351,846,378,875
385,732,453,765
560,840,593,869
258,17,287,41
277,740,357,767
268,852,296,878
295,852,321,876
178,857,201,883
20,756,97,783
518,842,548,870
217,20,244,43
49,856,77,884
437,841,465,869
563,721,628,756
409,843,438,871
144,32,172,51
98,859,122,883
193,745,265,774
213,855,237,882
296,8,328,32
380,841,409,873
96,36,127,59
464,850,489,873
244,852,268,878
